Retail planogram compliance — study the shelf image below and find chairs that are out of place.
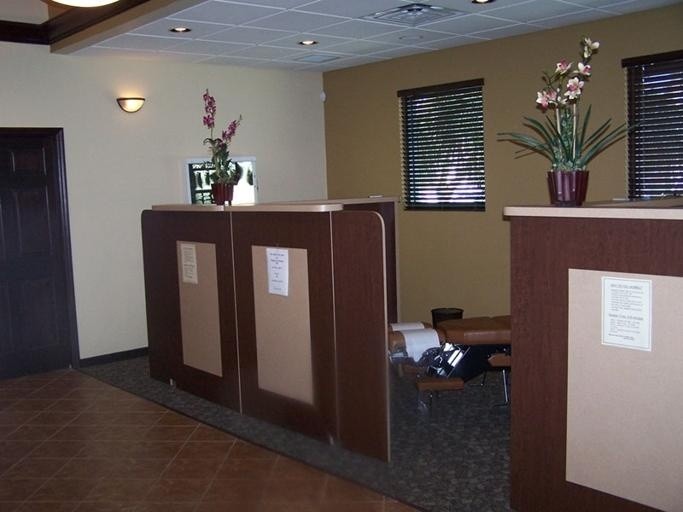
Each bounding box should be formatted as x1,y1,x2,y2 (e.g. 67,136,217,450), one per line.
388,312,511,416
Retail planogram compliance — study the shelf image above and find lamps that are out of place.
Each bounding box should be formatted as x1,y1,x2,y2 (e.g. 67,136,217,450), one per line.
117,90,144,113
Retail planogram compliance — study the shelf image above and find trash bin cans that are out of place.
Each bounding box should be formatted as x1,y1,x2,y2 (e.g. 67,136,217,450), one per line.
432,308,464,345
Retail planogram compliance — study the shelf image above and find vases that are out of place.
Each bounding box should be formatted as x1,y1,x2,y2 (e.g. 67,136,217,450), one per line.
546,166,588,205
211,180,230,202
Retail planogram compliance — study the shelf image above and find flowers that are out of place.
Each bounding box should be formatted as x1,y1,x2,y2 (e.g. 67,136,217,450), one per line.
491,26,641,169
196,85,248,184
